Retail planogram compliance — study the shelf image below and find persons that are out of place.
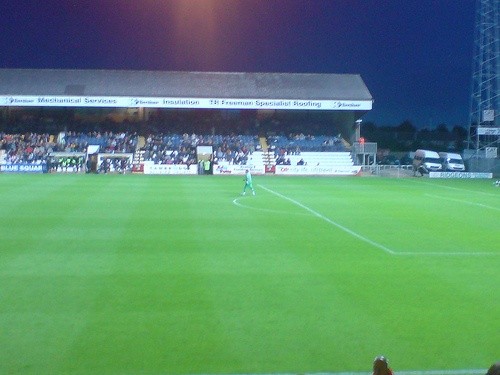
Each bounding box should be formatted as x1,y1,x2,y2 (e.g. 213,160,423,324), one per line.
0,131,343,175
243,169,255,196
417,162,429,176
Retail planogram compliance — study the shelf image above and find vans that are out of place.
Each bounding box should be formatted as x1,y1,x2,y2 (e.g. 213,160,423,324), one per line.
413,150,442,171
436,152,465,172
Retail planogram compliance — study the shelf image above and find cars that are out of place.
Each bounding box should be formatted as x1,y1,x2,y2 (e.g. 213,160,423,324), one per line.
366,154,413,169
